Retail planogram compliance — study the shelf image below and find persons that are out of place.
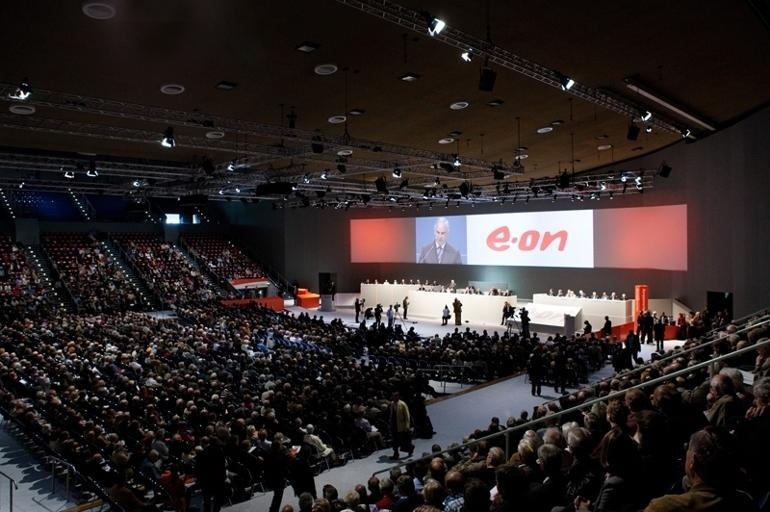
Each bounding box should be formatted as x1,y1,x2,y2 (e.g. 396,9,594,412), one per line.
0,218,769,510
